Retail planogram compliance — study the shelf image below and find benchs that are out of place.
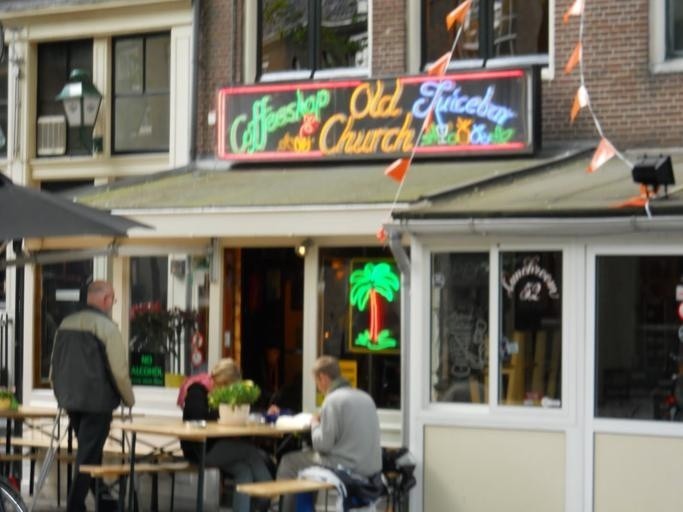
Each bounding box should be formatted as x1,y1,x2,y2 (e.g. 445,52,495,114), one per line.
0,438,401,512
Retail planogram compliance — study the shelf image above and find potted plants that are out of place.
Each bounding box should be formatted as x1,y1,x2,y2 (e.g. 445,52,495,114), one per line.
207,379,261,424
0,390,16,415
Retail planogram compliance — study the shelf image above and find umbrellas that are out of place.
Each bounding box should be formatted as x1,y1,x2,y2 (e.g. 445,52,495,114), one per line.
0,173,159,483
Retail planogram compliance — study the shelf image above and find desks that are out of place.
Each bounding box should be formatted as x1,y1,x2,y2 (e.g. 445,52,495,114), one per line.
0,409,143,512
109,417,312,511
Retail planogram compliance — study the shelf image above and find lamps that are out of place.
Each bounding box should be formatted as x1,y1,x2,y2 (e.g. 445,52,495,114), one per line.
55,68,103,155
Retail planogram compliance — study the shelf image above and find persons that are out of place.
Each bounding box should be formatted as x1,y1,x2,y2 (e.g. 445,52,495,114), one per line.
265,354,384,512
45,277,135,512
179,358,279,511
